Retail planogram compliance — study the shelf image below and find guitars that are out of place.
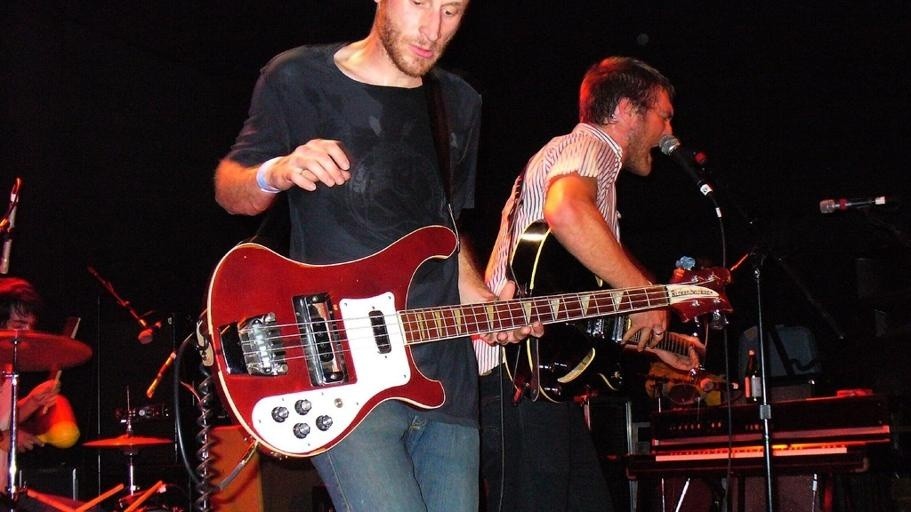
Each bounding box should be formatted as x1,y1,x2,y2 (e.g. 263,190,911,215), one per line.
205,225,737,461
675,262,735,290
499,217,729,406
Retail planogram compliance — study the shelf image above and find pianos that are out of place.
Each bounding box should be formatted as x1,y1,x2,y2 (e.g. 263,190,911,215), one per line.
650,389,895,478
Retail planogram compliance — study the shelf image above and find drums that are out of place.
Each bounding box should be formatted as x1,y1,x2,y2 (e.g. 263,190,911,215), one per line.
115,485,191,512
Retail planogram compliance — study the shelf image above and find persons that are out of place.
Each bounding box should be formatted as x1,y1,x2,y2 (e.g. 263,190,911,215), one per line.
0,278,62,506
213,0,544,512
474,56,707,511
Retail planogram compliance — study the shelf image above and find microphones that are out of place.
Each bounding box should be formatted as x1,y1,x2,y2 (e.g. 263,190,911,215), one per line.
819,196,887,214
145,352,178,400
0,193,19,275
138,320,164,346
657,135,715,198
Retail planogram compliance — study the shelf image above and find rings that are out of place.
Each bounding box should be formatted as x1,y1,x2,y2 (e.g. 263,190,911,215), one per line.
654,331,663,336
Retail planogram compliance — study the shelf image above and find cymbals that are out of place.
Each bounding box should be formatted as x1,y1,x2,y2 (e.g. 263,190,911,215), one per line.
81,434,174,449
23,487,97,511
1,329,93,373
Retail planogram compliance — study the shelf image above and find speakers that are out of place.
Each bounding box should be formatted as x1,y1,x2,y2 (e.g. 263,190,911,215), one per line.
727,466,820,512
783,378,784,379
739,324,824,378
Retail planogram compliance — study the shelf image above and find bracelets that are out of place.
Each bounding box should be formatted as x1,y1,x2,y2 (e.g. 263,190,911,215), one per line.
255,155,284,194
